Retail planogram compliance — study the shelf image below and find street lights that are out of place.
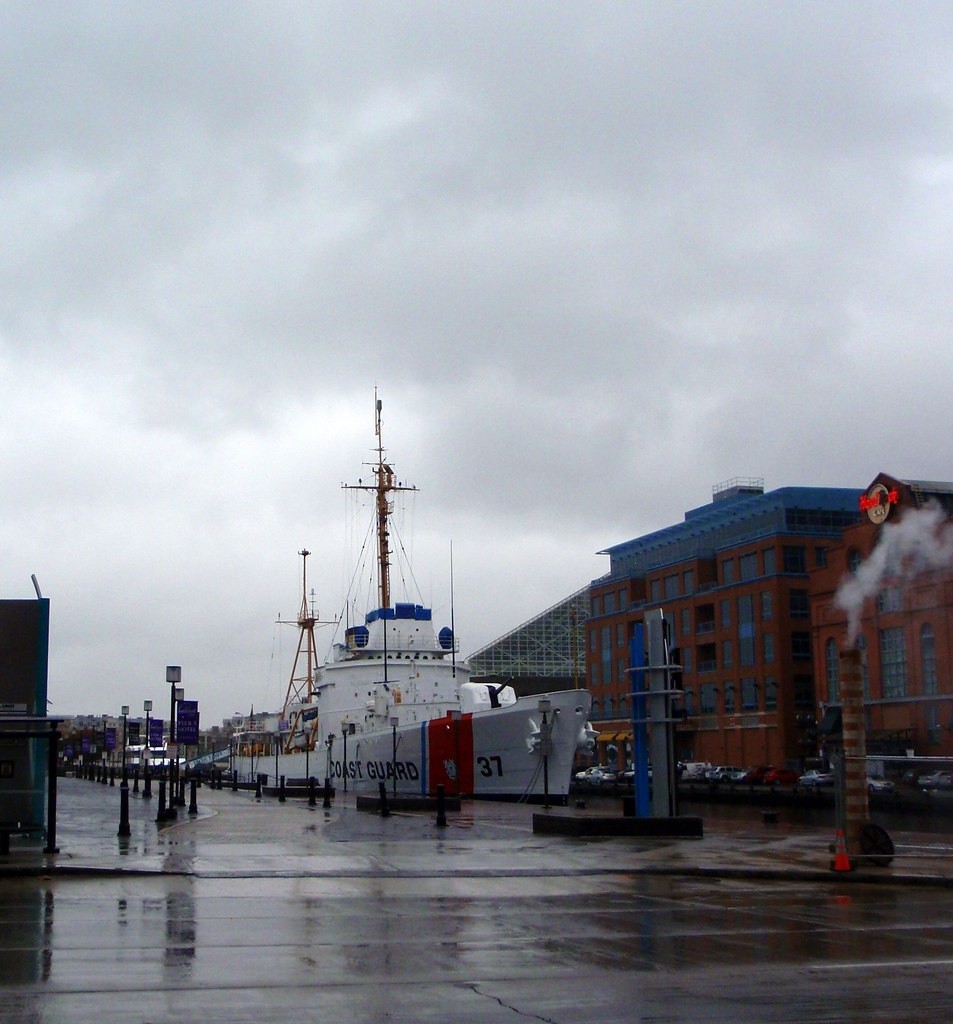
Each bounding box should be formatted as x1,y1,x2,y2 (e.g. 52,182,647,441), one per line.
117,705,129,837
156,665,181,821
142,700,152,798
101,714,108,785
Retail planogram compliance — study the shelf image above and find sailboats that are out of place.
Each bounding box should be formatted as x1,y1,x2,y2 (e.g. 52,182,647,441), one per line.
227,385,592,805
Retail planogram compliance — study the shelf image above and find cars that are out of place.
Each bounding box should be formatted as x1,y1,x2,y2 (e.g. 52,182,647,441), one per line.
617,761,800,788
865,775,895,794
903,767,938,788
925,769,953,789
587,767,616,783
797,769,836,787
575,767,601,781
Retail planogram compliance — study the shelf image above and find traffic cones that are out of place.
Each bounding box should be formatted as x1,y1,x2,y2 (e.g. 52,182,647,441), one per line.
830,827,852,872
